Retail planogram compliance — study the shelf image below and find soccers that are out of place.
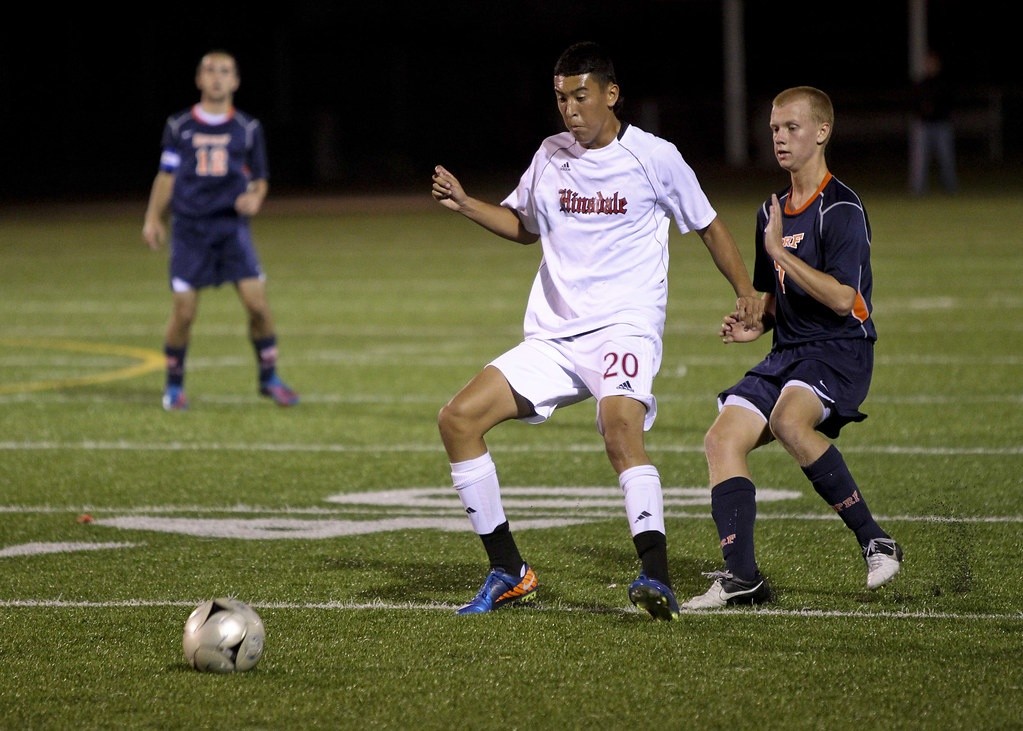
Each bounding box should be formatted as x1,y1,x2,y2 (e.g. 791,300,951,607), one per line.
181,596,268,675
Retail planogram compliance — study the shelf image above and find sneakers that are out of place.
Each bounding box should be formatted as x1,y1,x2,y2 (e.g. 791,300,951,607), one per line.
455,562,538,614
678,569,771,614
628,570,680,621
861,535,903,591
162,386,187,411
260,374,298,406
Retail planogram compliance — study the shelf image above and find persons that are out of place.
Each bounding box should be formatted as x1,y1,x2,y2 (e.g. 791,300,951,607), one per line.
431,41,763,622
142,49,298,411
679,88,903,609
